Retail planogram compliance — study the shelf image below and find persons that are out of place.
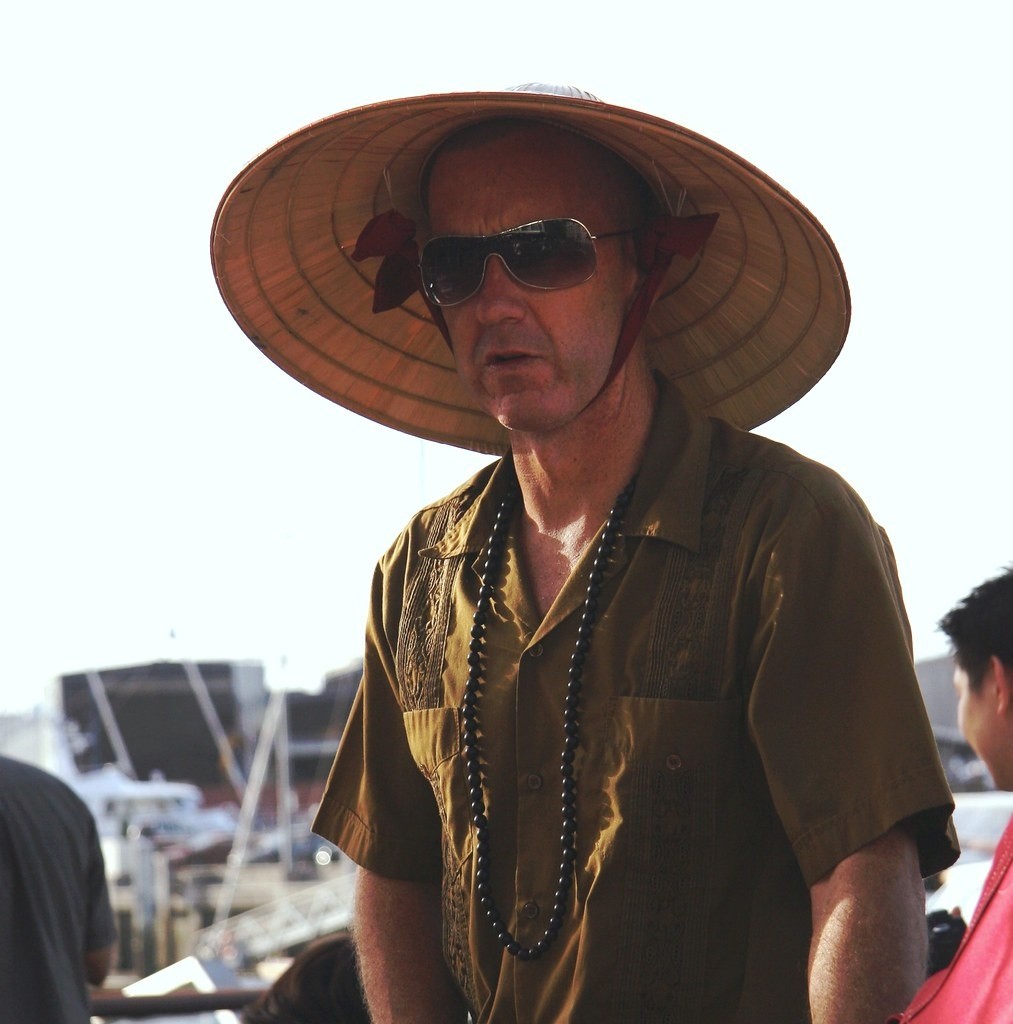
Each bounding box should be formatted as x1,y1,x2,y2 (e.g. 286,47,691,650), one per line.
208,82,963,1024
887,572,1013,1024
0,757,118,1024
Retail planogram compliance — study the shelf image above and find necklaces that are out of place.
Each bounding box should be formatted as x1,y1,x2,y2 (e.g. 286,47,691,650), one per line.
463,473,635,960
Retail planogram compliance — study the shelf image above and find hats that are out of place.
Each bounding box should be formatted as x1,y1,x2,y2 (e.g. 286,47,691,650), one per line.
211,82,851,455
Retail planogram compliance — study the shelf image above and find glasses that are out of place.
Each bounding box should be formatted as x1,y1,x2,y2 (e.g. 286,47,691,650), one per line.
416,218,636,306
